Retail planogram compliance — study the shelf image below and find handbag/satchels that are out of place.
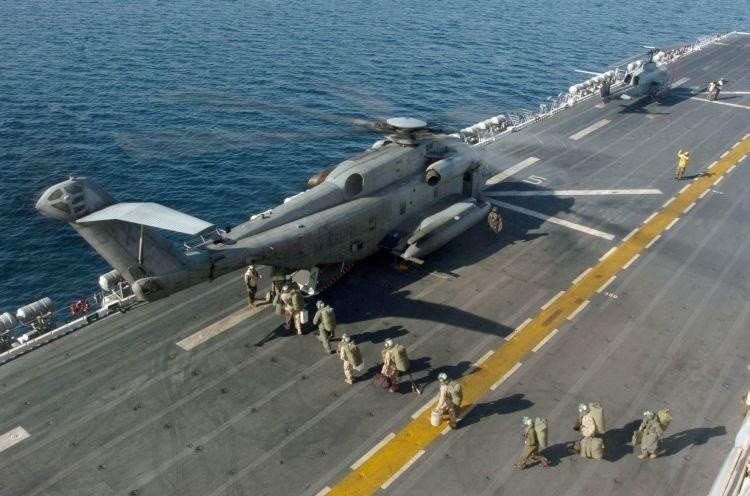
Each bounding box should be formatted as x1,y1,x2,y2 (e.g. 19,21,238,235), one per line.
573,417,583,431
578,437,605,461
372,372,391,389
633,429,644,446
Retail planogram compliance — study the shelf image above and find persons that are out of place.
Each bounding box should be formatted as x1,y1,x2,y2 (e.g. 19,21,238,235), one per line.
337,332,356,385
714,75,726,99
243,265,260,302
312,299,337,354
705,78,717,101
673,148,690,180
570,402,597,454
434,371,457,427
513,414,552,470
265,273,307,336
636,409,664,459
380,336,405,393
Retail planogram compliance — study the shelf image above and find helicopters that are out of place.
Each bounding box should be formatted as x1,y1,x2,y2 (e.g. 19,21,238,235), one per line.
33,79,621,323
599,40,693,104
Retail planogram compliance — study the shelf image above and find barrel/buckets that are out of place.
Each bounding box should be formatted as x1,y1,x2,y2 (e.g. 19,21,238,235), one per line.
300,310,309,324
353,357,364,372
431,409,443,426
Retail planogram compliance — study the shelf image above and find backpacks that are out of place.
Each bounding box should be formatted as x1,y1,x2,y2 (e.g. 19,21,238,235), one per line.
657,407,673,433
393,344,410,372
292,290,305,313
320,304,337,333
448,381,463,409
587,402,606,436
534,417,548,449
345,341,363,367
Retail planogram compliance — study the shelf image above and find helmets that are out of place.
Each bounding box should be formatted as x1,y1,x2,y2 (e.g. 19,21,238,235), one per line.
282,285,290,292
316,300,325,309
521,416,534,427
437,372,447,383
644,411,655,421
342,332,350,342
577,403,589,414
384,338,395,349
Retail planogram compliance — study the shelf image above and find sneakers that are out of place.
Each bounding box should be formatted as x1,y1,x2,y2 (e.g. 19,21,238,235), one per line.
344,378,355,386
283,323,303,336
